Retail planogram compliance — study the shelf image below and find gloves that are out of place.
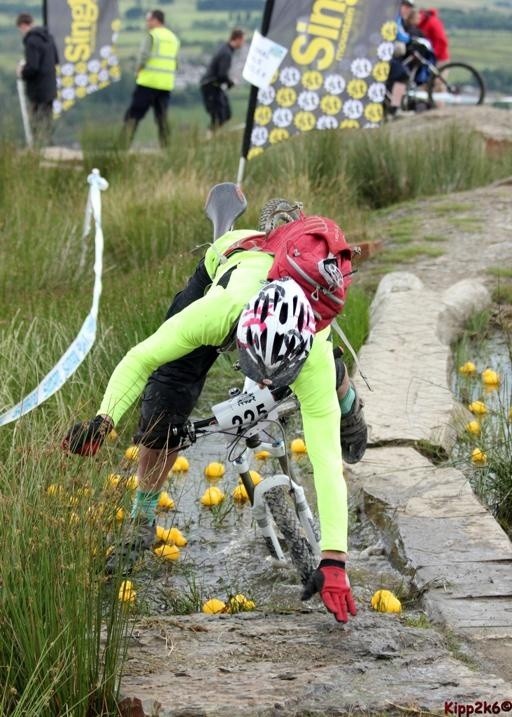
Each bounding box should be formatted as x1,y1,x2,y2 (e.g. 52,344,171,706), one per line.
61,414,114,458
300,559,360,623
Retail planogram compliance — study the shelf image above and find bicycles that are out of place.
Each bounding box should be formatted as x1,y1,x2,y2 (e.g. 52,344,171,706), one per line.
171,181,373,589
385,36,485,117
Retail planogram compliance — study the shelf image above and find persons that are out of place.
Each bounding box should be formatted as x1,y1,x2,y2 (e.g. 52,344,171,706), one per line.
200,29,247,127
124,9,180,149
401,1,448,97
16,10,60,149
385,1,412,120
62,229,367,628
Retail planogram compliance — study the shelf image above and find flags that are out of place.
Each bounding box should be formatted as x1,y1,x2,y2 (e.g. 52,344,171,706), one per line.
46,0,122,118
249,0,398,159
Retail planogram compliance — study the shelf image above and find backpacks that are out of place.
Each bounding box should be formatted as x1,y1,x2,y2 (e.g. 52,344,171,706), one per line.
264,216,353,330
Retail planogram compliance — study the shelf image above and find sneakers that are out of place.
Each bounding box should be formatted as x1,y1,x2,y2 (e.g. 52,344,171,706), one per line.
339,384,367,466
107,519,156,578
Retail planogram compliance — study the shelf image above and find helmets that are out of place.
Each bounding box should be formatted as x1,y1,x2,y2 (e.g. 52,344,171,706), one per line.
238,277,317,388
